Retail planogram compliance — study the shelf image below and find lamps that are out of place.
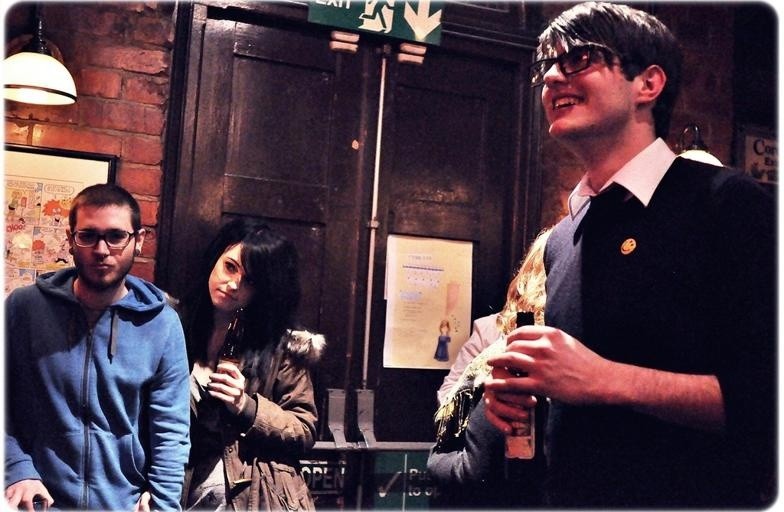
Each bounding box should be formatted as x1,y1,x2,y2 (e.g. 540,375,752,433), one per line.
4,1,79,107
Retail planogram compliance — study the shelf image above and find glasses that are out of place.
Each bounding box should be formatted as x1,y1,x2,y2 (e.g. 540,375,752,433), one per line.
69,228,142,250
526,42,620,92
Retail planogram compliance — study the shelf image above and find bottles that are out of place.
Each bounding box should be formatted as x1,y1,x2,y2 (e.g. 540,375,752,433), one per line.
502,311,547,471
207,307,247,410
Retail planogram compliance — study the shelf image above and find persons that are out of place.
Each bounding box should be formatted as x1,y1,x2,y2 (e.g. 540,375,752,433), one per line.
436,265,521,405
138,216,325,511
426,224,553,509
483,3,772,507
5,185,190,510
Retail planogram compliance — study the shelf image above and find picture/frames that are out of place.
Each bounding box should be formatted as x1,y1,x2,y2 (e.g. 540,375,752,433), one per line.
3,142,119,300
